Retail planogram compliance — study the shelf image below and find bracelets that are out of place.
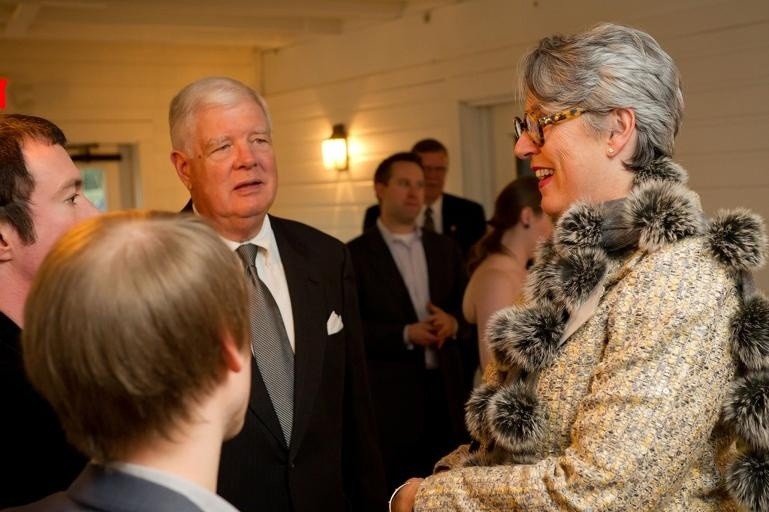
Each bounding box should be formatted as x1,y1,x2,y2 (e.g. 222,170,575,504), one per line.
388,482,410,511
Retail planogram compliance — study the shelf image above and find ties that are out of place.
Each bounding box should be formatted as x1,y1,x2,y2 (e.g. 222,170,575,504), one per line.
233,243,295,448
423,207,434,232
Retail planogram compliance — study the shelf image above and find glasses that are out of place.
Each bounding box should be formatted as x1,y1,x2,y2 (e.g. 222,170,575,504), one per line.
512,106,586,148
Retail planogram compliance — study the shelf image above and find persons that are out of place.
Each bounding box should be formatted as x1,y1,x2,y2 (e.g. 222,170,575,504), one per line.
16,208,265,511
349,155,460,399
386,22,769,512
462,177,562,396
0,113,116,507
165,74,367,507
364,136,492,278
